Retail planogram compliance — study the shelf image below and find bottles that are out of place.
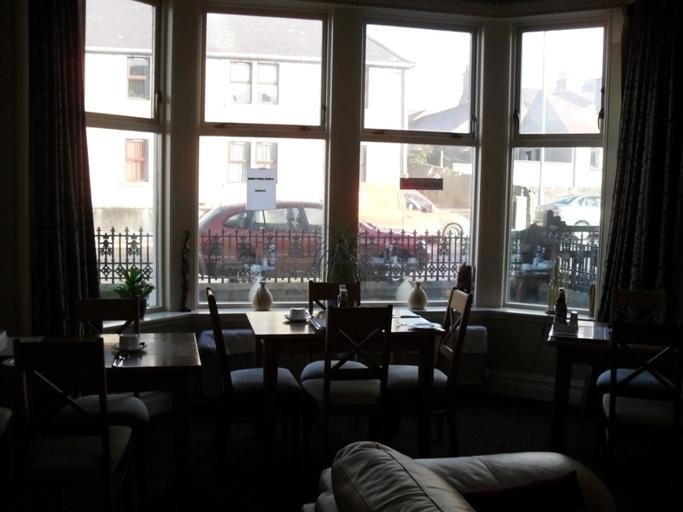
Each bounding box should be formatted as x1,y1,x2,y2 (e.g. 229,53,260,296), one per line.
336,283,348,308
546,264,560,312
407,280,426,309
569,311,577,331
247,277,260,303
554,286,566,325
395,276,412,302
251,281,273,312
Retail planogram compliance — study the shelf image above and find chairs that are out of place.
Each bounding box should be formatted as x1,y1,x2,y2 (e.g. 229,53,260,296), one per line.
1,249,682,510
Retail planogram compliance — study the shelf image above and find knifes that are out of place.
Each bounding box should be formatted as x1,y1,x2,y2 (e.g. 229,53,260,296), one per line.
112,353,118,367
392,315,421,318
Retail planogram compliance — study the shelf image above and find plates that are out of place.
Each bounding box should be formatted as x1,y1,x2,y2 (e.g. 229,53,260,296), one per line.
285,314,311,322
110,342,147,355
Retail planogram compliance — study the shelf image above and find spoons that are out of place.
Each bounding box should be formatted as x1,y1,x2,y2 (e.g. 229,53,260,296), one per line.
117,352,128,367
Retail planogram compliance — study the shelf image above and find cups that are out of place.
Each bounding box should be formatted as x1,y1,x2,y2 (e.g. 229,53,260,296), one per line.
119,335,139,350
288,306,305,319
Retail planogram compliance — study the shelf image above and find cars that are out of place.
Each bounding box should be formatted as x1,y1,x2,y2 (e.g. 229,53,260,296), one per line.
537,194,600,240
198,199,432,283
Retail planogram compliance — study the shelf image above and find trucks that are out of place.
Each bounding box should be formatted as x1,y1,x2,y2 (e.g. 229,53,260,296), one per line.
358,181,472,242
